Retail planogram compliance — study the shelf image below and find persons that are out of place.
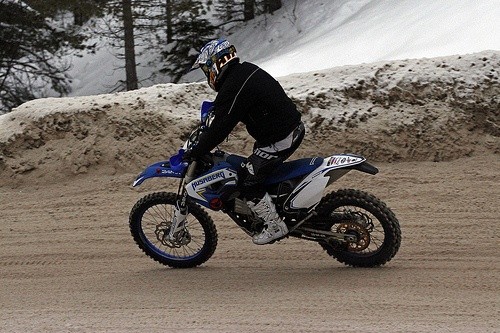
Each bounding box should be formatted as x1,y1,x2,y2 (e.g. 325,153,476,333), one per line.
180,39,305,245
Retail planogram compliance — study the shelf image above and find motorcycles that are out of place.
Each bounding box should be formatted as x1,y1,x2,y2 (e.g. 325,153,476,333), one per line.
130,101,401,267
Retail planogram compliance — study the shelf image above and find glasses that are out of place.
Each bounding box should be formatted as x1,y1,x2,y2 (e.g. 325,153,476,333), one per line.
201,65,208,77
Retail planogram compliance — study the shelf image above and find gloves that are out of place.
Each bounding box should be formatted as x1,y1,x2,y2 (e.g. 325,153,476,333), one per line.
182,146,200,164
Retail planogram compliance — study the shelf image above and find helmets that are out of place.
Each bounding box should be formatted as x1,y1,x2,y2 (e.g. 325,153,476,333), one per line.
190,39,239,92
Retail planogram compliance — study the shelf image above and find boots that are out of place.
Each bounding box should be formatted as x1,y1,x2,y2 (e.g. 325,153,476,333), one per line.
247,191,288,245
